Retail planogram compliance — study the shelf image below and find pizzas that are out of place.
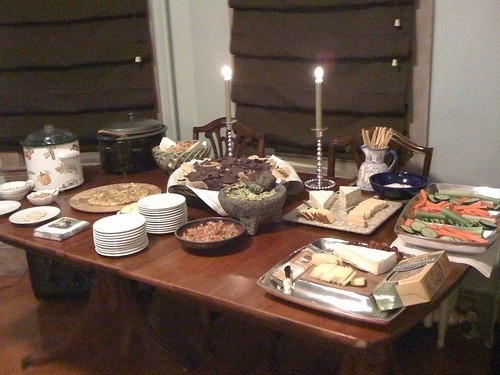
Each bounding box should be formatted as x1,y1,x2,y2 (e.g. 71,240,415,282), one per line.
88,184,152,206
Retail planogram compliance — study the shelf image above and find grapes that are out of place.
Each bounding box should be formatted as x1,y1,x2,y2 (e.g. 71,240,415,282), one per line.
348,239,404,264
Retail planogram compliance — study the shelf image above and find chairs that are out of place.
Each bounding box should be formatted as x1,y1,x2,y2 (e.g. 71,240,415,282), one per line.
327,127,434,185
193,117,264,160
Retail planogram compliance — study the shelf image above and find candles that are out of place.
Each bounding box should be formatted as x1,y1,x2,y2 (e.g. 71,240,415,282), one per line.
222,64,233,122
313,67,324,128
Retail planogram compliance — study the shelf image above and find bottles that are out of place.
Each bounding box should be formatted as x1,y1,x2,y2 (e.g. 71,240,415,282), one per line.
356,145,397,192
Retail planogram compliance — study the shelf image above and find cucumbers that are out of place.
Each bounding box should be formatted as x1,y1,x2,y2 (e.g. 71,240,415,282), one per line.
475,219,497,230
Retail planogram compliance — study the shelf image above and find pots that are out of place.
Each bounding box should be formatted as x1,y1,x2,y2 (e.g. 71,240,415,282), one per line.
93,113,168,174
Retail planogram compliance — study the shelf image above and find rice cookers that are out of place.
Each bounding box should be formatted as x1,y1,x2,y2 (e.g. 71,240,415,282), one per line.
20,126,85,192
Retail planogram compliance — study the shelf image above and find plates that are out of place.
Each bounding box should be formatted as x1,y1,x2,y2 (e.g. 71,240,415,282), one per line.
69,183,162,213
8,206,60,225
282,193,403,234
257,237,407,323
174,217,245,251
92,192,188,257
393,182,500,254
0,201,22,215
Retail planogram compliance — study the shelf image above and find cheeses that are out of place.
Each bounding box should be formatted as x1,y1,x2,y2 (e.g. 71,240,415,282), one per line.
333,243,397,275
309,191,335,209
340,186,361,208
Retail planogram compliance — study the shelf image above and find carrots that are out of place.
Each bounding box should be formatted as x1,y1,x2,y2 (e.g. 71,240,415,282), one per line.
414,189,490,218
405,218,488,243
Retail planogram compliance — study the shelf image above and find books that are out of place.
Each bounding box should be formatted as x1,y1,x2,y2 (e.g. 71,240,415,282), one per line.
33,216,92,242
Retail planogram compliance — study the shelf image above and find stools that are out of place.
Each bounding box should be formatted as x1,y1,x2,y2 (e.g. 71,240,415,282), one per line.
425,265,500,348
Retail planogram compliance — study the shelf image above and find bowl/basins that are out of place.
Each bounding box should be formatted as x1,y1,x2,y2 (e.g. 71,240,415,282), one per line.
0,181,30,201
26,190,59,205
370,171,427,200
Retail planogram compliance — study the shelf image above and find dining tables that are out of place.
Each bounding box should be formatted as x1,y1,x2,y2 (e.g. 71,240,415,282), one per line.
0,160,470,375
